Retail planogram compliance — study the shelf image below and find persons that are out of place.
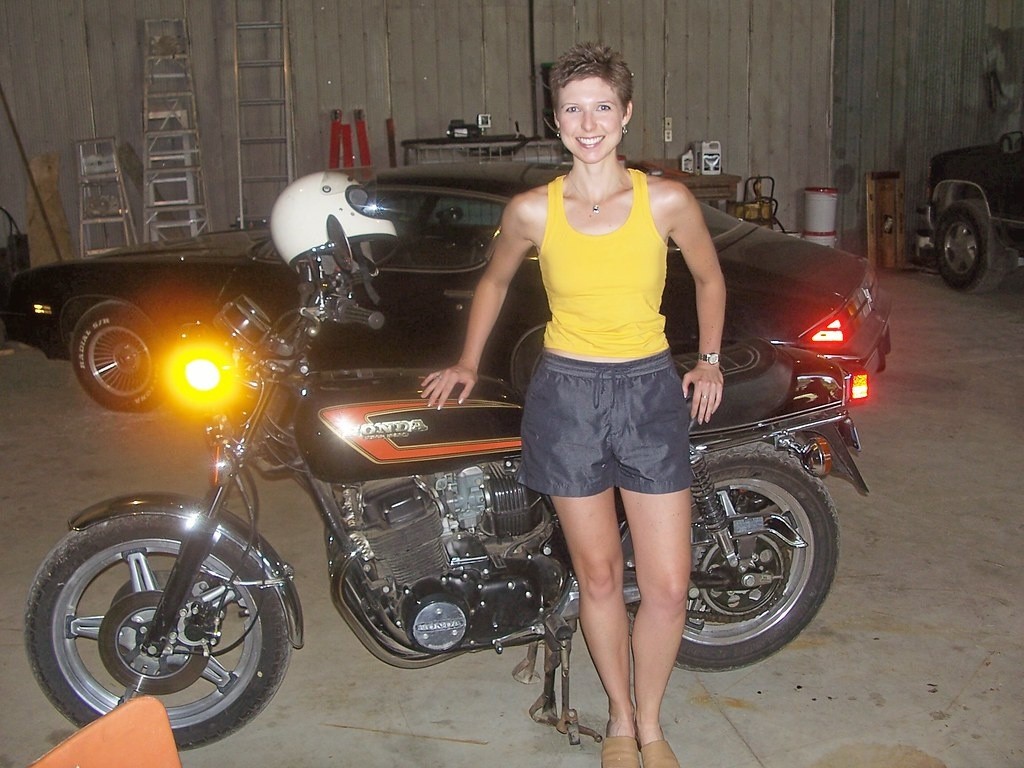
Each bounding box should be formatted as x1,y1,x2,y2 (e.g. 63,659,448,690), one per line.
420,40,728,767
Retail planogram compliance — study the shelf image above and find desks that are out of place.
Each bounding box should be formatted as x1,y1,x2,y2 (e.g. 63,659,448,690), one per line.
629,159,741,218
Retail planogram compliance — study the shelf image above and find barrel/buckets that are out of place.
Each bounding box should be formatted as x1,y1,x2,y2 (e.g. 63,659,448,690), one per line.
802,187,838,232
804,230,837,250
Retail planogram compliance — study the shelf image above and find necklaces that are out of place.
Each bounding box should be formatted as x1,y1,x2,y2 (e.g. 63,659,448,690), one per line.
568,174,600,214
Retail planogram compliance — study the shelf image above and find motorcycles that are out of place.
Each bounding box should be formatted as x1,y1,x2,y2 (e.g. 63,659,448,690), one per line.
25,210,871,745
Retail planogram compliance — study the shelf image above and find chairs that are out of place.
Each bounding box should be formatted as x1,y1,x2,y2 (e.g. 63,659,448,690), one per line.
24,695,183,768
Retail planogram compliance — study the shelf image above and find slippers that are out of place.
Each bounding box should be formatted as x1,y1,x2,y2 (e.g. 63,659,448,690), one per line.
601,720,642,768
636,727,679,768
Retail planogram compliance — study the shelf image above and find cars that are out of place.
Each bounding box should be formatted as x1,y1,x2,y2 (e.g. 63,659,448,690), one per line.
6,159,894,415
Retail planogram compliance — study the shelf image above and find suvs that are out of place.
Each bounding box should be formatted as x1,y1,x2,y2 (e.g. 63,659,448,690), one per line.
929,132,1024,294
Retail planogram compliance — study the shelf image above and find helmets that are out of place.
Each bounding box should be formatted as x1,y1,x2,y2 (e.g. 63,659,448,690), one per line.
271,171,399,271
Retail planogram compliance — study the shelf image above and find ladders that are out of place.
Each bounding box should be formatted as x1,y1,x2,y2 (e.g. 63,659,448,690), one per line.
232,0,296,230
75,137,139,260
142,17,211,243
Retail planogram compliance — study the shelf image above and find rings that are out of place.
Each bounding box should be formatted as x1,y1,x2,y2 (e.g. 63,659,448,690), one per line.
437,375,441,380
702,395,708,398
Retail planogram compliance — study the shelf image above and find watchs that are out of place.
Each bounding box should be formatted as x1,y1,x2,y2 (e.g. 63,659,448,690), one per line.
696,352,722,365
